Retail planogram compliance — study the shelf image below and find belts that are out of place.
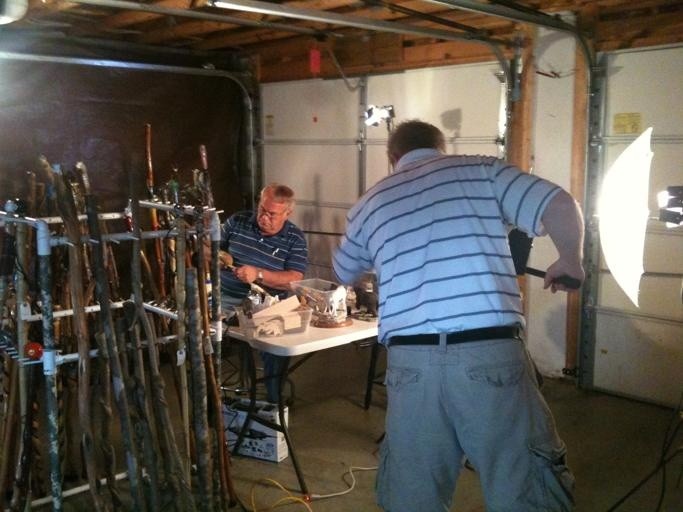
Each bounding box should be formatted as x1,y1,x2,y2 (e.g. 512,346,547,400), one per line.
387,322,521,349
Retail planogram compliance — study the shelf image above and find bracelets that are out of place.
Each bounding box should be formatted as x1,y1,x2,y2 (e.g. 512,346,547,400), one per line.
255,268,264,285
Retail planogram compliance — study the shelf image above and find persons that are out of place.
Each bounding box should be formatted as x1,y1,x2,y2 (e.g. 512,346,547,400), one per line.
331,121,586,512
203,183,309,403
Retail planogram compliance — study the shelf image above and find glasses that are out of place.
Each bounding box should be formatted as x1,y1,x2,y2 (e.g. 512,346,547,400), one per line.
257,203,287,218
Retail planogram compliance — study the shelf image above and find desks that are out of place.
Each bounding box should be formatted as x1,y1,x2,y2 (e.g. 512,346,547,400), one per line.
224,301,384,501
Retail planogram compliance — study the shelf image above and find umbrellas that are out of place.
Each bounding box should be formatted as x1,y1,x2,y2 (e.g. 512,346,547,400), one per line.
593,126,658,308
